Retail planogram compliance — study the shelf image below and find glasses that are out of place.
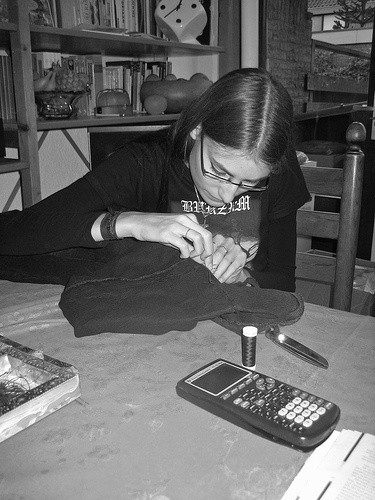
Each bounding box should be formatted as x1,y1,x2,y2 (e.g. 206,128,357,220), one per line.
199,131,270,192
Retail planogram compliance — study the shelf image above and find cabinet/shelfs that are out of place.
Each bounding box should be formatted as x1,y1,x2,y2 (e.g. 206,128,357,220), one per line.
0,0,242,211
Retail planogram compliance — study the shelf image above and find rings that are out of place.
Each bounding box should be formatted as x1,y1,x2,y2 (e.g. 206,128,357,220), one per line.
213,242,217,247
219,246,228,252
185,227,190,238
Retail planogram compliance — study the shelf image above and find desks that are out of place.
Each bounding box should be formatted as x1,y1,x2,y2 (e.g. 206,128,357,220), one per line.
0,279,375,500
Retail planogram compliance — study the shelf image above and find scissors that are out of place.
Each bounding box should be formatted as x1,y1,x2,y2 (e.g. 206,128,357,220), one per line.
259,320,329,370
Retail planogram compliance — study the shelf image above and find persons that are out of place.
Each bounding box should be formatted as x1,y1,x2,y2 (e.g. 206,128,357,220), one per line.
0,69,313,294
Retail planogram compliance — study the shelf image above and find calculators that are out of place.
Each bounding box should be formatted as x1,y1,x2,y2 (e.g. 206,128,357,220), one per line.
175,359,341,453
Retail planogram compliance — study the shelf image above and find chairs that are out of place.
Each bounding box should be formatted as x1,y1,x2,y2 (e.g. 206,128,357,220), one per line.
295,122,367,312
